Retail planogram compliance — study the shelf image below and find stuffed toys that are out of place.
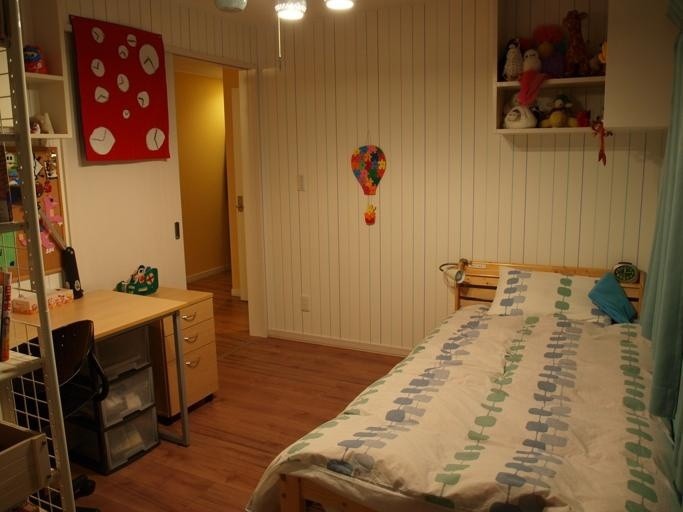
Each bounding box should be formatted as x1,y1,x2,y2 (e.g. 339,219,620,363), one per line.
503,9,614,166
23,42,49,75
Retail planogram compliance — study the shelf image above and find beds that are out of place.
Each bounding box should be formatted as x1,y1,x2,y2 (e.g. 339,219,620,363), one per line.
281,262,681,511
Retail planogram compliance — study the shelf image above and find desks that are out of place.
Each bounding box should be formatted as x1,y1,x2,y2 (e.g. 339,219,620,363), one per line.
0,290,191,450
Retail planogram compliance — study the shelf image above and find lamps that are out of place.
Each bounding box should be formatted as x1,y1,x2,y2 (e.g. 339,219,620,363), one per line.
208,0,359,63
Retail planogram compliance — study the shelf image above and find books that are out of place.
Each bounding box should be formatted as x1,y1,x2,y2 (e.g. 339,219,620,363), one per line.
0,271,12,361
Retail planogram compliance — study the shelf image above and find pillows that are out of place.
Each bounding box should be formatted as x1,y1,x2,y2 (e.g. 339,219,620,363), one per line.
485,265,616,327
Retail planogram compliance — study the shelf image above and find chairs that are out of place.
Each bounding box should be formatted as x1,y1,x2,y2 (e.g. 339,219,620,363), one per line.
15,319,109,499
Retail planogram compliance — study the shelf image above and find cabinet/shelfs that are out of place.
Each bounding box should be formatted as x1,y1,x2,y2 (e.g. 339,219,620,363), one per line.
148,286,218,420
488,0,676,135
0,2,76,511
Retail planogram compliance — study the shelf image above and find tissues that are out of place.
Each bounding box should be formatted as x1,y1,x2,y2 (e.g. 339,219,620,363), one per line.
11,287,75,315
